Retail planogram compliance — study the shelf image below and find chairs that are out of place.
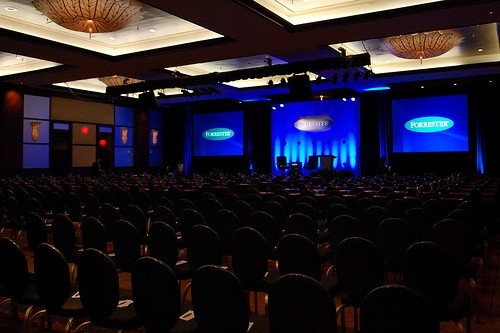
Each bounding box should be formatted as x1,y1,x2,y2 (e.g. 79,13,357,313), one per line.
0,168,500,333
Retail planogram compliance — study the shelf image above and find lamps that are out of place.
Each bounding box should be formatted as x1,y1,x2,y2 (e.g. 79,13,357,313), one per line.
158,92,169,100
315,76,321,85
332,72,338,84
354,71,361,80
269,80,273,85
181,86,220,97
266,57,272,66
364,70,372,80
99,75,145,87
31,0,144,33
339,47,346,56
342,71,350,82
380,31,464,64
281,78,287,88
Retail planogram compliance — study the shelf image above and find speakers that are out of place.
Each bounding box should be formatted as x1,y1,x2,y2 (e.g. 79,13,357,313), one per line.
287,75,312,102
139,92,158,109
